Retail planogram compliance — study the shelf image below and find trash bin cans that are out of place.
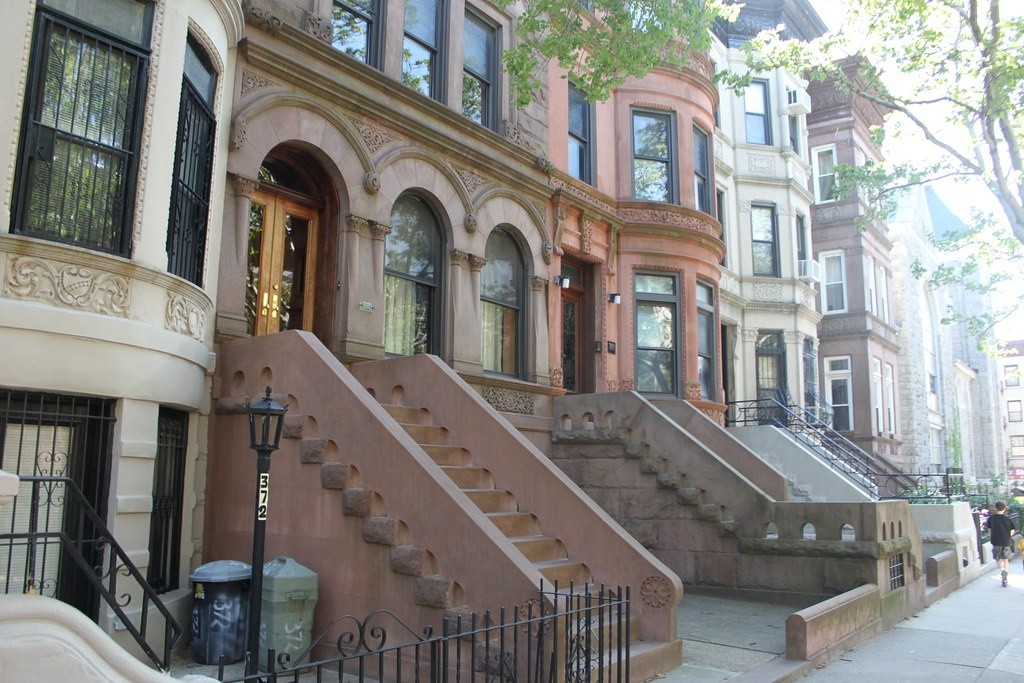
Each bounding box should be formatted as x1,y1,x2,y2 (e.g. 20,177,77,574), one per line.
189,559,252,665
260,555,318,678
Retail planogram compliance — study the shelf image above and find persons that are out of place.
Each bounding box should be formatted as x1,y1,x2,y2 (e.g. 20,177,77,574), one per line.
983,500,1015,588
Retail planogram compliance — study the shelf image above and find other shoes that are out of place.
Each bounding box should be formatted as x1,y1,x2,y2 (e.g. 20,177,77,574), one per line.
1001,571,1008,582
1002,580,1007,587
